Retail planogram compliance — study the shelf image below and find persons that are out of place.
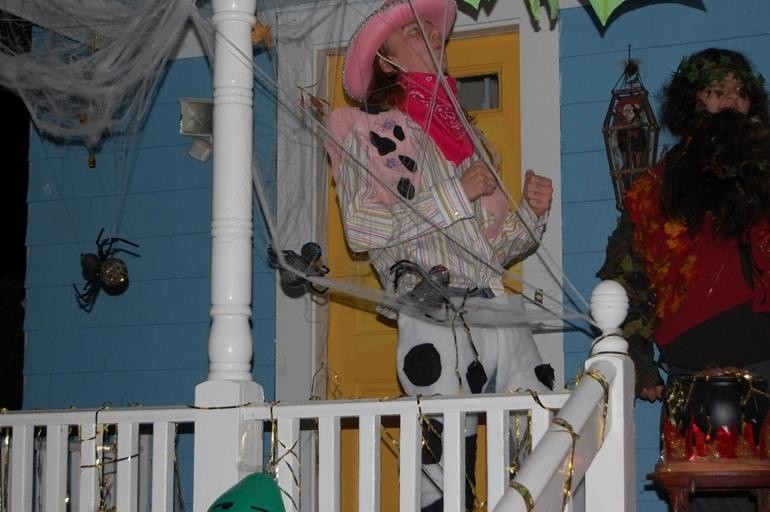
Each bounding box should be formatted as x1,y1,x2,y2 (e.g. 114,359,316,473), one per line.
596,49,770,403
323,1,554,512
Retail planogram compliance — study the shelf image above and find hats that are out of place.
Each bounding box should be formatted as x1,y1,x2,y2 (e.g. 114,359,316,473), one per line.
343,0,457,101
